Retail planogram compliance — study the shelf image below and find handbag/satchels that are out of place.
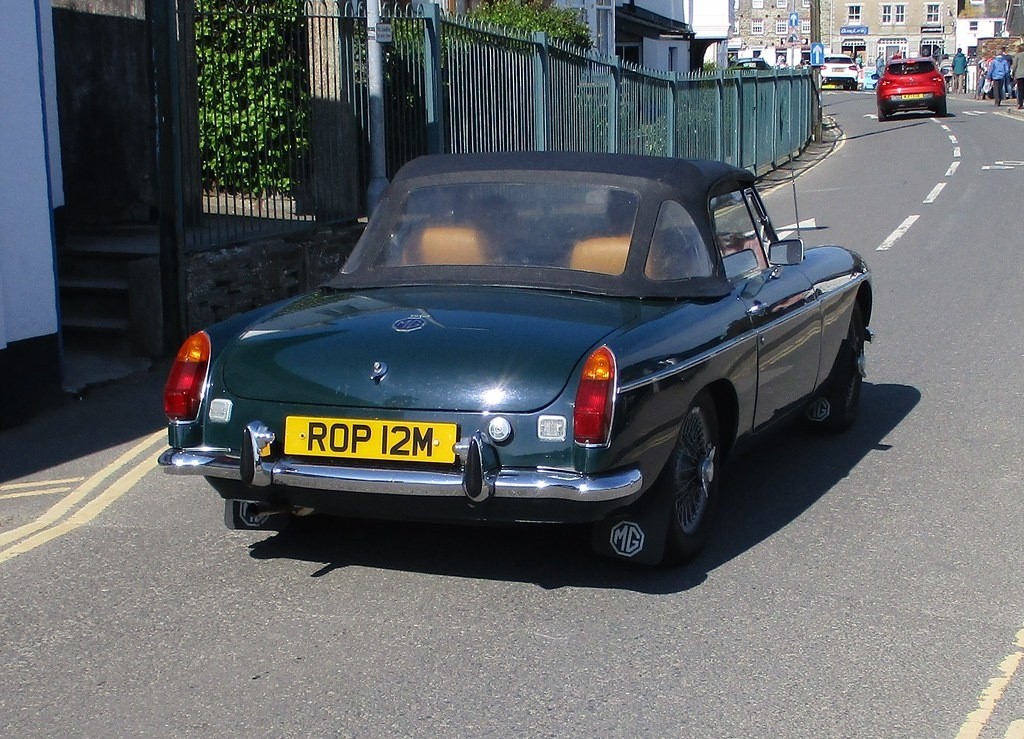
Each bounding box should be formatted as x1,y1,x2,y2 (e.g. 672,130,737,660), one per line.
982,79,993,94
871,73,880,80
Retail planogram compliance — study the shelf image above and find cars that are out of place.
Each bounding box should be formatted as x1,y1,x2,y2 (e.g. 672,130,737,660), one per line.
795,60,811,70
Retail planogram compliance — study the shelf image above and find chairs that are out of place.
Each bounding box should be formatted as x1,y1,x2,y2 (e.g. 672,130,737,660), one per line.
403,227,506,265
570,235,669,282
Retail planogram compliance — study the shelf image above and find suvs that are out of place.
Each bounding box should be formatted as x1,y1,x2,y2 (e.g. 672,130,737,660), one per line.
734,58,771,70
820,54,859,89
870,56,950,120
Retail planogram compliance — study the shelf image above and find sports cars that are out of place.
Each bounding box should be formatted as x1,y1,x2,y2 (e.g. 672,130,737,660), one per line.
156,148,876,551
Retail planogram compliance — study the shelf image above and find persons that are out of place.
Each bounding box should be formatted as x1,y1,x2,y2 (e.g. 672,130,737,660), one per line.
606,189,666,238
471,192,519,246
777,44,1024,110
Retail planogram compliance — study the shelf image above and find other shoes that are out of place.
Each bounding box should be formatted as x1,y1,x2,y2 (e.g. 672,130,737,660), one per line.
1017,105,1024,109
